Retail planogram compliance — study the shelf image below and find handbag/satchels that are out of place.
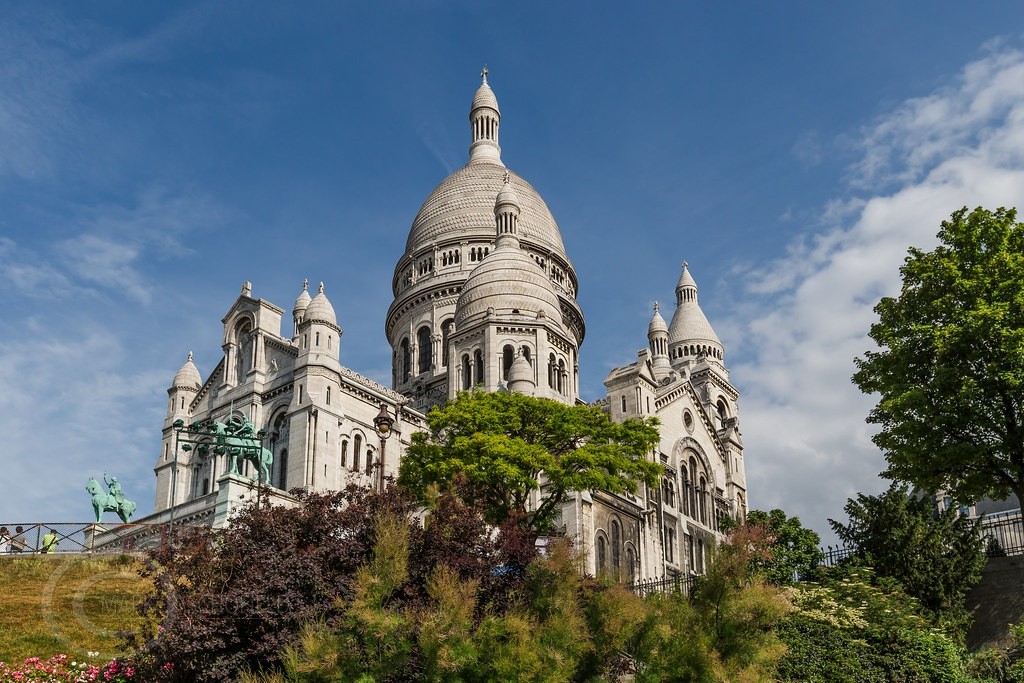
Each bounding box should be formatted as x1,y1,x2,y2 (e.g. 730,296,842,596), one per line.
41,547,48,554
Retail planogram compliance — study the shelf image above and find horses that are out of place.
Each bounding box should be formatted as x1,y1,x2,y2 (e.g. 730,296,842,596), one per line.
85,475,137,523
214,422,273,485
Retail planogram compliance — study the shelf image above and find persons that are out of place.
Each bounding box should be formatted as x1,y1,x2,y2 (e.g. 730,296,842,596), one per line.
0,527,14,555
12,526,27,554
230,415,256,453
42,529,59,554
104,472,121,509
